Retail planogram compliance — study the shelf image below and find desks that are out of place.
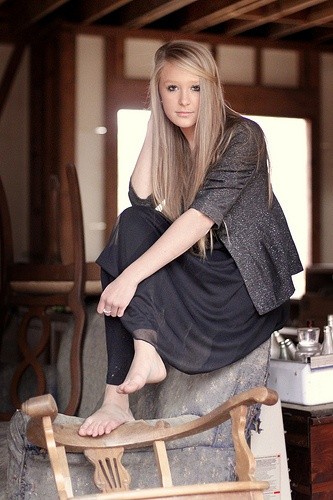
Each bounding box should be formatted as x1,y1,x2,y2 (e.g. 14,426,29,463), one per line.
281,401,333,500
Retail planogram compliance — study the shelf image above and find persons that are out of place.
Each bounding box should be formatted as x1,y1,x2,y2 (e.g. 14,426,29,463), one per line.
79,38,304,437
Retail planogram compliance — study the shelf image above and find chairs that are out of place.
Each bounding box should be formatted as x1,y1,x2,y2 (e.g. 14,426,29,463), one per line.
21,386,279,500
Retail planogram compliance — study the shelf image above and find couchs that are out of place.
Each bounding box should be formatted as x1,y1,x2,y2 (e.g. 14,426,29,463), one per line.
0,302,271,500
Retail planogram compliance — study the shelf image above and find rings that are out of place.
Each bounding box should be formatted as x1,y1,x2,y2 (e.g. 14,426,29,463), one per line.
103,309,112,314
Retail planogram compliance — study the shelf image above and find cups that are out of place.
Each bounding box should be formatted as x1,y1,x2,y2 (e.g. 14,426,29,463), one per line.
297,327,320,357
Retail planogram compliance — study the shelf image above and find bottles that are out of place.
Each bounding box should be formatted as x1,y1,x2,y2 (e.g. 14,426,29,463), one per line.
321,315,333,355
